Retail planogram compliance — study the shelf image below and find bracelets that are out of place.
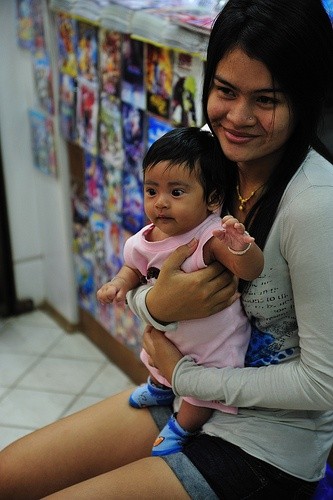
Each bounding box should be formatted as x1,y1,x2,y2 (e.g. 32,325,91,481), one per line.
228,231,252,255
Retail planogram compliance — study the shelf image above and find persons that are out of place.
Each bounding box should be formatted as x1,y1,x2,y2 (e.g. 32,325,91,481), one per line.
0,0,333,500
96,127,265,456
